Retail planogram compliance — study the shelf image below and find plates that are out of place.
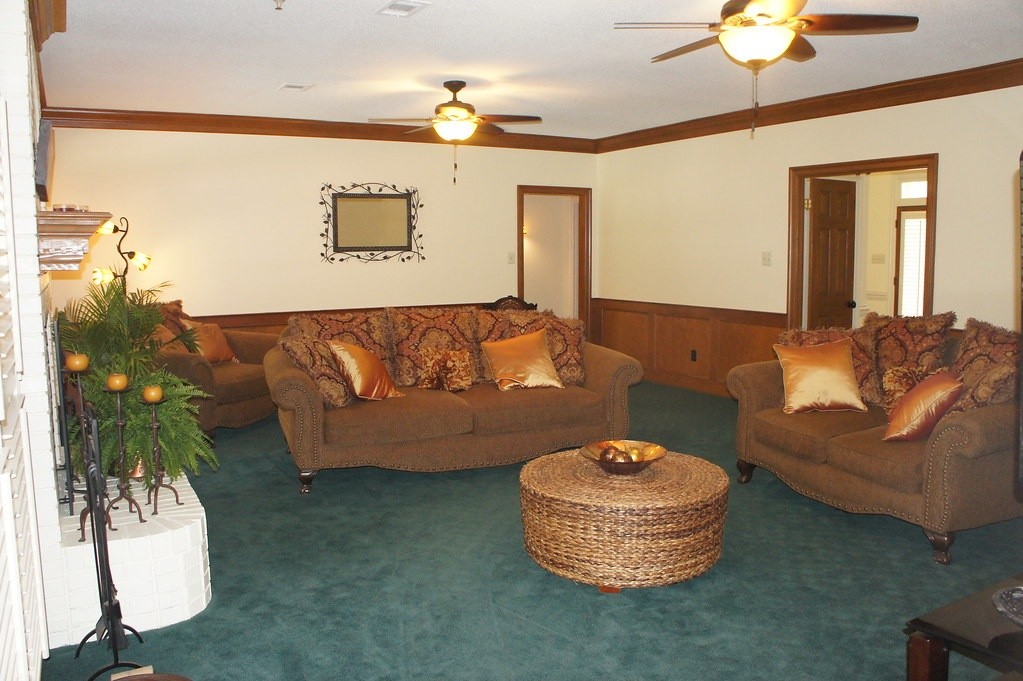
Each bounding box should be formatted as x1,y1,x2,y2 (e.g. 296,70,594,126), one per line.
580,440,666,474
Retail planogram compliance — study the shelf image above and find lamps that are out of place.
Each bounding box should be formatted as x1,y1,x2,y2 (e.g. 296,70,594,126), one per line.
613,0,919,141
91,216,152,296
368,80,542,183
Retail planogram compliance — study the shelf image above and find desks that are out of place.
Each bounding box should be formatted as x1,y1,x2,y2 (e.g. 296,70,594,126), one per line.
901,571,1023,681
518,448,730,594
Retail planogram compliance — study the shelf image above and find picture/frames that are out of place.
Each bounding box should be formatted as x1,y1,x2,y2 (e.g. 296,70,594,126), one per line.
332,192,412,252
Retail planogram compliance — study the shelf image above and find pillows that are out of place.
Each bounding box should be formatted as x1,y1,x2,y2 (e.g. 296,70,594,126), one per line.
773,311,1023,441
139,300,237,365
276,305,586,410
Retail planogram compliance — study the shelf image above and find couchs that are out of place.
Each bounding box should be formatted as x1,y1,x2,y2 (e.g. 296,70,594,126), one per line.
262,338,645,494
142,329,280,441
725,357,1023,564
